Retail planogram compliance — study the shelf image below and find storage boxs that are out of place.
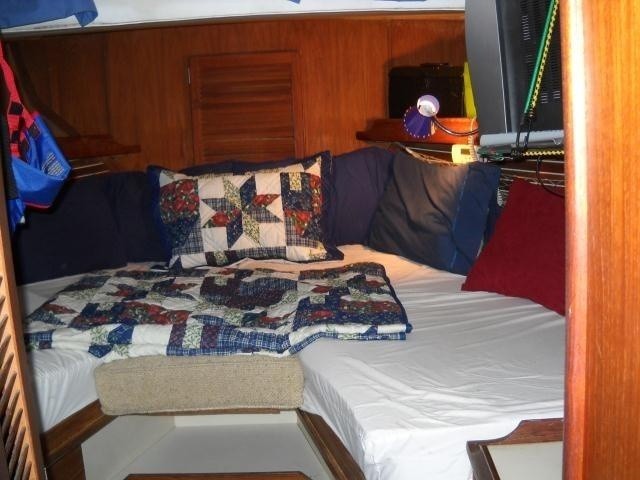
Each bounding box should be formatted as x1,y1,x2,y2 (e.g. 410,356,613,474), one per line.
388,63,467,117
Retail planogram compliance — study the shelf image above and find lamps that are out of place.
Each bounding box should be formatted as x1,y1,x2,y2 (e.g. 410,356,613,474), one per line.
402,95,479,140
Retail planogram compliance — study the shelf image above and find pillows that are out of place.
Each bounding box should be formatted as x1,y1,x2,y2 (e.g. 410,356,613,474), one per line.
11,175,128,285
458,176,566,317
111,170,169,263
333,143,394,244
363,148,501,276
146,150,344,270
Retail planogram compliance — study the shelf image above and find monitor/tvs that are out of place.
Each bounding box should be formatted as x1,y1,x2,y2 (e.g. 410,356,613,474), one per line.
465,0,563,146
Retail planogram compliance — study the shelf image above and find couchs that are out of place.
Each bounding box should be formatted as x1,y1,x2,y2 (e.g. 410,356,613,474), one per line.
0,141,565,480
